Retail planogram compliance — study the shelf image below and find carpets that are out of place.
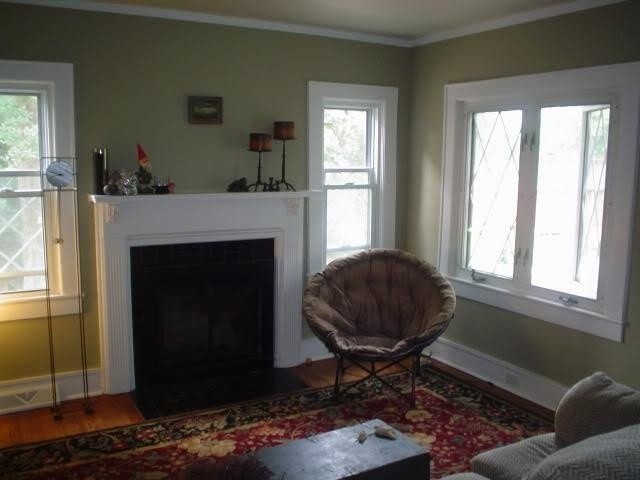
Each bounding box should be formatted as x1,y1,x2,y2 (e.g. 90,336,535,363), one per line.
0,365,555,479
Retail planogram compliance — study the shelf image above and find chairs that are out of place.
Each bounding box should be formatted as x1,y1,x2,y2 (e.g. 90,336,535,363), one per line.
301,248,457,409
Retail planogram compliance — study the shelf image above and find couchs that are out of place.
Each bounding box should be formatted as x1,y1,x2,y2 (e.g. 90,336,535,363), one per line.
442,370,640,479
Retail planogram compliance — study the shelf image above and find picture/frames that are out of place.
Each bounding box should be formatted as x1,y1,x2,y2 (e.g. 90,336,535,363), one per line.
187,95,223,126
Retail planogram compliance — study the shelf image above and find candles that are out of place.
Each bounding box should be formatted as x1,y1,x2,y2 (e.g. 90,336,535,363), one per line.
249,121,295,150
